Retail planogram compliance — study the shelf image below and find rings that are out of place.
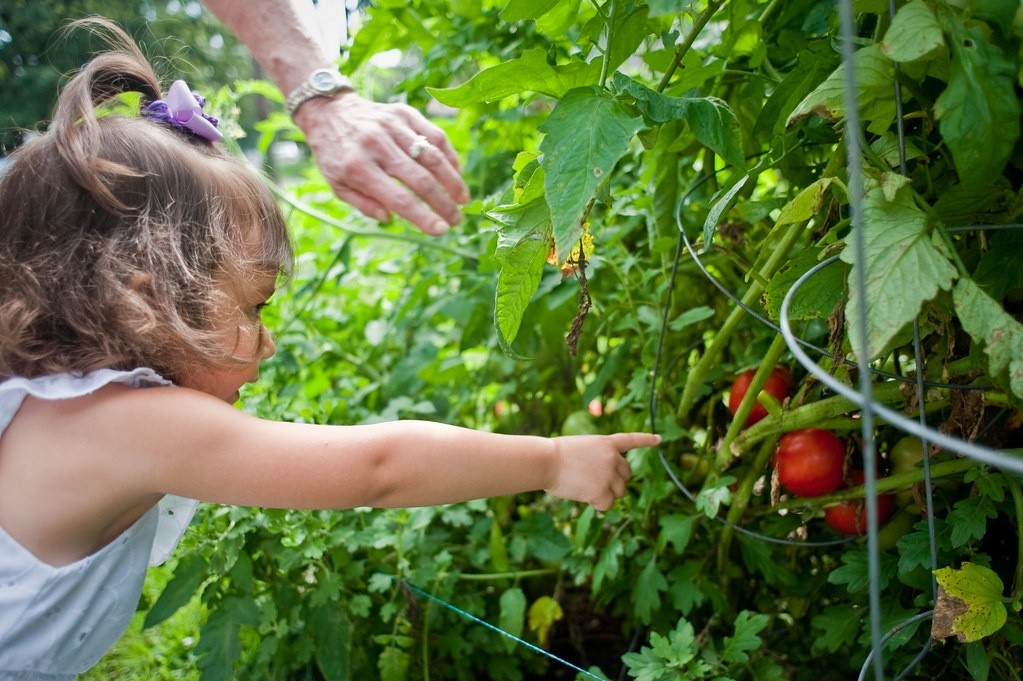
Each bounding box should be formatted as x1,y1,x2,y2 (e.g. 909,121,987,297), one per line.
407,136,427,157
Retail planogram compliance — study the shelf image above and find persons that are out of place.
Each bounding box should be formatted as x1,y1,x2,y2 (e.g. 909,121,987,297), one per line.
0,50,664,681
200,0,469,232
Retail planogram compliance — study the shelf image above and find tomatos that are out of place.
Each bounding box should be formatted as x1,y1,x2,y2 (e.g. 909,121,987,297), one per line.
729,368,942,531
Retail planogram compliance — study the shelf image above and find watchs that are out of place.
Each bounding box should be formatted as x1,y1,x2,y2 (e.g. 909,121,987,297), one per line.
285,68,350,121
